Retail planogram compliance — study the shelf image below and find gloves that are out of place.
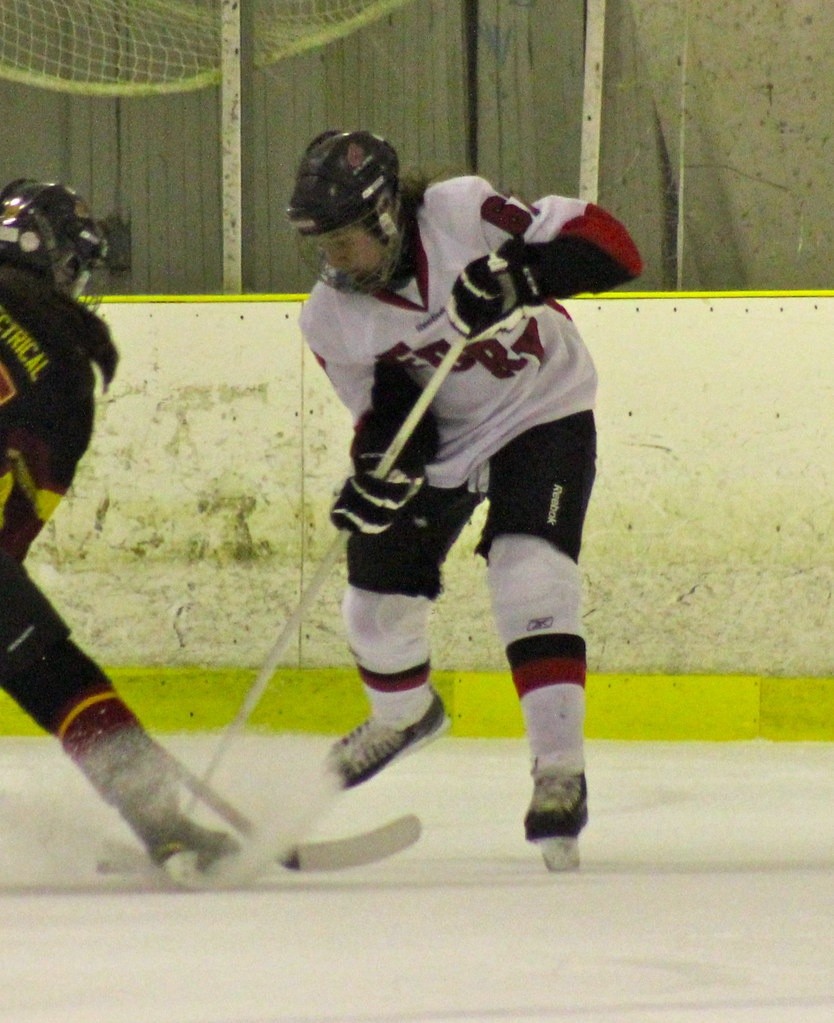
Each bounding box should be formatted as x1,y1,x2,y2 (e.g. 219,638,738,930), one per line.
445,242,582,340
330,416,426,537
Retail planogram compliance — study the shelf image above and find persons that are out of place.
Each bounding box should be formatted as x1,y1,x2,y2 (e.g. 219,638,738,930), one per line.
0,174,268,886
282,128,644,873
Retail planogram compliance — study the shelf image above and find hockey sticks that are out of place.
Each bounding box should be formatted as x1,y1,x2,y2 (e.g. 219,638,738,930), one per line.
140,733,418,872
94,235,525,875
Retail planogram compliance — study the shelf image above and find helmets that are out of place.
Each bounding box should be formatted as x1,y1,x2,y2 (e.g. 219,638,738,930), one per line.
285,130,402,297
0,179,110,300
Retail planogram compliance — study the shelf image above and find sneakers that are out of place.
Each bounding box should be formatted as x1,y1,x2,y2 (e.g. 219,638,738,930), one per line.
522,767,587,872
320,688,452,792
148,820,259,885
